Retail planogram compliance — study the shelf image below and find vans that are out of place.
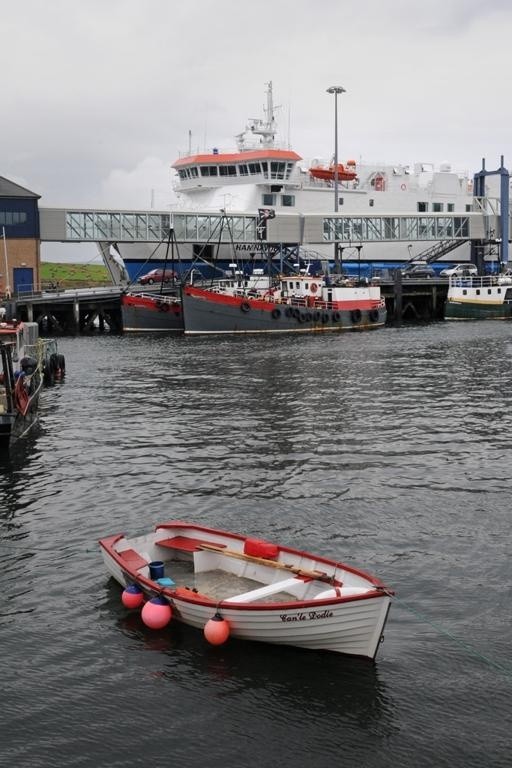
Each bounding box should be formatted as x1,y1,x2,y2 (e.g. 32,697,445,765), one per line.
440,263,477,276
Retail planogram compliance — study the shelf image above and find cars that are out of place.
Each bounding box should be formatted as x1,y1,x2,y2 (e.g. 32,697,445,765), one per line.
392,264,435,278
501,269,511,277
138,268,177,286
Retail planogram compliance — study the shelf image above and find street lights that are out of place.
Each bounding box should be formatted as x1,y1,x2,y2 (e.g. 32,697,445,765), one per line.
323,83,346,273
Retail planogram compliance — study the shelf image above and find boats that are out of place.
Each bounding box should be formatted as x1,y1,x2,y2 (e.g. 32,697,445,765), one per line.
0,307,65,387
443,272,512,321
181,209,388,336
1,340,46,461
120,210,186,333
91,82,512,285
97,521,394,667
